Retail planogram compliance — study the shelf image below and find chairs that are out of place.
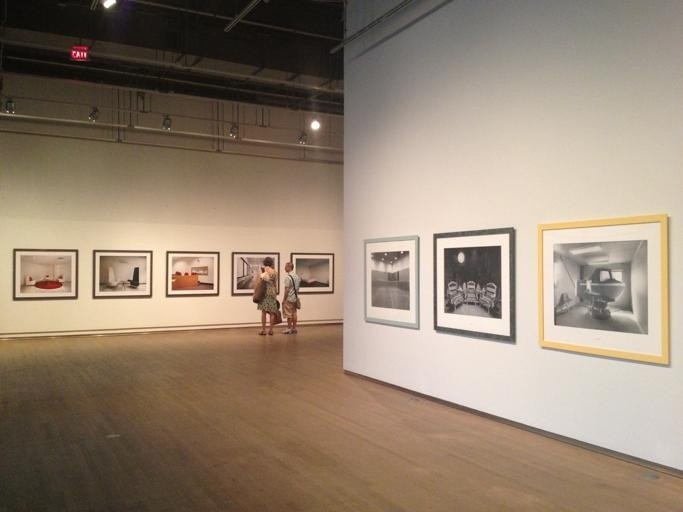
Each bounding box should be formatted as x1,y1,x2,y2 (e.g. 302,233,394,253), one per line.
107,267,118,287
56,274,64,282
128,267,139,287
447,280,497,313
25,276,36,286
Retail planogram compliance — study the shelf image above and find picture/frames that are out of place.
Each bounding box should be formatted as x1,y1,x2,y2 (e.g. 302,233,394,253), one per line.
291,252,334,294
93,250,152,299
363,235,419,330
231,252,280,296
13,249,78,301
538,214,671,367
432,227,515,344
166,251,220,297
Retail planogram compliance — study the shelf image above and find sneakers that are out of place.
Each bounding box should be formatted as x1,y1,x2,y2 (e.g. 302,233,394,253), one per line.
258,331,273,335
282,327,297,334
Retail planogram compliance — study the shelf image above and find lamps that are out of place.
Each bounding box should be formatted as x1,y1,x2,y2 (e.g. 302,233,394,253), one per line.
0,94,308,145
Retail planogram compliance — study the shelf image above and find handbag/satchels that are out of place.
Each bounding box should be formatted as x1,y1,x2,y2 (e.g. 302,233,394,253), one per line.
273,311,282,325
252,278,266,303
296,297,301,309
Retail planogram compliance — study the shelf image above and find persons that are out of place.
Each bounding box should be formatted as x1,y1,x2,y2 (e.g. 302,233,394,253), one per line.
281,262,301,334
254,257,278,336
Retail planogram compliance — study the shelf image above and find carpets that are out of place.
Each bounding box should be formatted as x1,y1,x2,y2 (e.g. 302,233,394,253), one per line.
36,281,63,289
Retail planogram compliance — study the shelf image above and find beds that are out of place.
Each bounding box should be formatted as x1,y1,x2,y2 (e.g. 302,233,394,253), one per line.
120,280,127,290
